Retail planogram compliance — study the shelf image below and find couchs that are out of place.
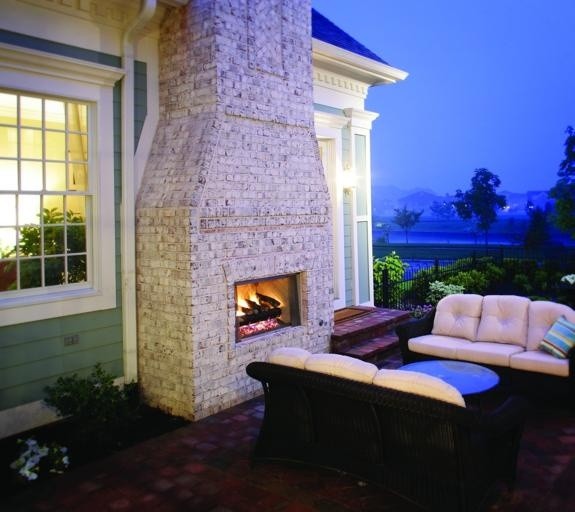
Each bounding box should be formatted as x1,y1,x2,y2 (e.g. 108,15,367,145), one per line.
394,289,575,423
241,344,524,511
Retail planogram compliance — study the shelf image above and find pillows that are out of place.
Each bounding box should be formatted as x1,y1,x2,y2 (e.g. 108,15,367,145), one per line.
538,312,574,358
